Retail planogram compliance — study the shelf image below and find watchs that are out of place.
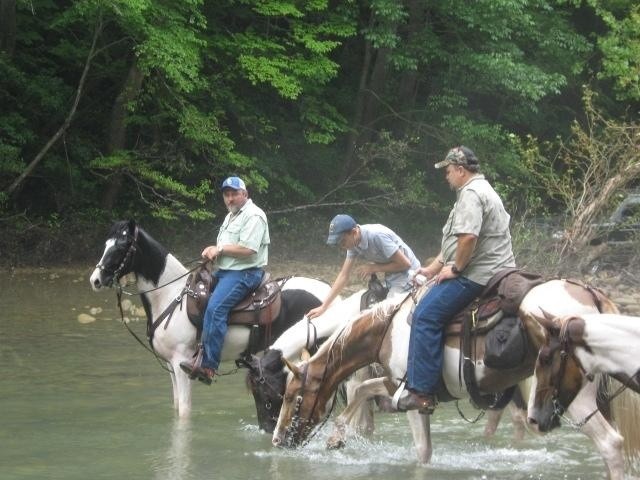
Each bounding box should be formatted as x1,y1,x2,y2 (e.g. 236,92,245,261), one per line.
451,263,462,276
217,244,224,256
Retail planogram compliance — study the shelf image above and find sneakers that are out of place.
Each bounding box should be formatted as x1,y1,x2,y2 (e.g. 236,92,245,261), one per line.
389,390,436,416
179,361,215,386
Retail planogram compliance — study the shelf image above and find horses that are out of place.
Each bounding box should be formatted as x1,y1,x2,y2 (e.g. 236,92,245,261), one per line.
234,266,640,480
90,217,348,411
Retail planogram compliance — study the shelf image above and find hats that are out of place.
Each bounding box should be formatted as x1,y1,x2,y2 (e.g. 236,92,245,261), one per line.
220,175,247,192
434,145,480,170
326,213,357,247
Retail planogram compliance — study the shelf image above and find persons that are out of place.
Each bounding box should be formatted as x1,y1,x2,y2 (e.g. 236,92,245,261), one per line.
305,213,423,321
381,145,516,414
178,175,270,386
